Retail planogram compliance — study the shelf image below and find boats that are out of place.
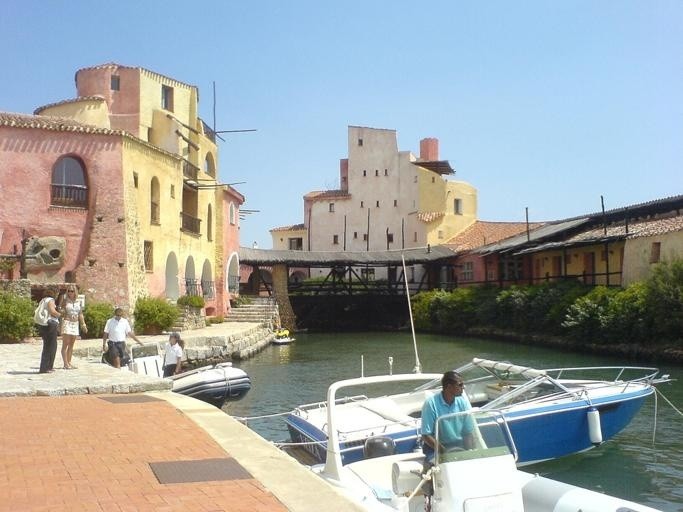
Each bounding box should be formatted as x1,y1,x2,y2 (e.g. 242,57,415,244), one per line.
271,336,296,345
102,342,251,409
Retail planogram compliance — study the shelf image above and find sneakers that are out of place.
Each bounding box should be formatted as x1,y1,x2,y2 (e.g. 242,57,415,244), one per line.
40,367,58,374
62,362,80,370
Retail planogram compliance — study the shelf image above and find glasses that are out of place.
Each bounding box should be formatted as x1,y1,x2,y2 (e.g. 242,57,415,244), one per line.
456,382,464,387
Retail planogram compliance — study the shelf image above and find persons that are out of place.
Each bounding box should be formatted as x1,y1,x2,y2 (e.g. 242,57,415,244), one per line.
102,308,145,368
57,283,88,370
274,320,281,330
161,332,185,378
35,284,64,374
419,370,475,497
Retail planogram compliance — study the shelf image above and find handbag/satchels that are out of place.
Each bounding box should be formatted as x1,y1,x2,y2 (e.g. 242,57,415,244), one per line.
31,300,51,326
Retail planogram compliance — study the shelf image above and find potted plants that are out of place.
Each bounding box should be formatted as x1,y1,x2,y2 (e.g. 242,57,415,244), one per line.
133,295,181,335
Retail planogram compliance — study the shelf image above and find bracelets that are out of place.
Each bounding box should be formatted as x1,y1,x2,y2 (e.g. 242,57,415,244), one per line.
80,321,86,327
103,345,105,347
